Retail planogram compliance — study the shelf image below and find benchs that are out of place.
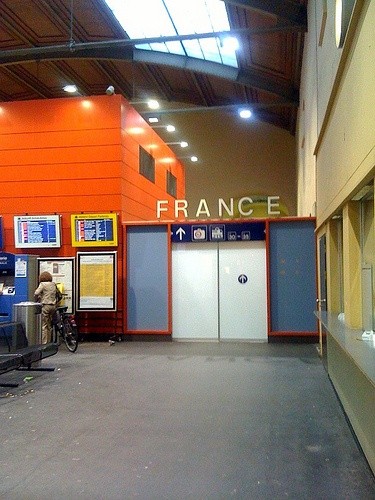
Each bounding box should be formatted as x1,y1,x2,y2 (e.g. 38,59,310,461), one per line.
0,322,58,388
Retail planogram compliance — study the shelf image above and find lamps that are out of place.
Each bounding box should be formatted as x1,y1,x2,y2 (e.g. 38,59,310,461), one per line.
129,100,159,109
62,84,82,97
166,142,188,147
152,126,175,132
106,86,115,95
179,157,197,162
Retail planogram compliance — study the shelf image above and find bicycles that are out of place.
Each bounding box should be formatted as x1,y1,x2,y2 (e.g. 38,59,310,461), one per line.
36,293,81,351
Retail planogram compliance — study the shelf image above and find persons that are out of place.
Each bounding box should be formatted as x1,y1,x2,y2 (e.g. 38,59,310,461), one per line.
33,271,62,344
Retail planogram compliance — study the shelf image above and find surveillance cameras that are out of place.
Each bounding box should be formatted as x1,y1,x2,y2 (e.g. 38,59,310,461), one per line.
106,85,114,96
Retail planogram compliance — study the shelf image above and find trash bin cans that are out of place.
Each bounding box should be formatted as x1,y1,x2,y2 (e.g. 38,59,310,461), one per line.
11,299,44,370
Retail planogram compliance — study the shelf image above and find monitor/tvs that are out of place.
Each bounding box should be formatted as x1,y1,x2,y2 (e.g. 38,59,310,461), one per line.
70,213,119,247
13,215,62,249
